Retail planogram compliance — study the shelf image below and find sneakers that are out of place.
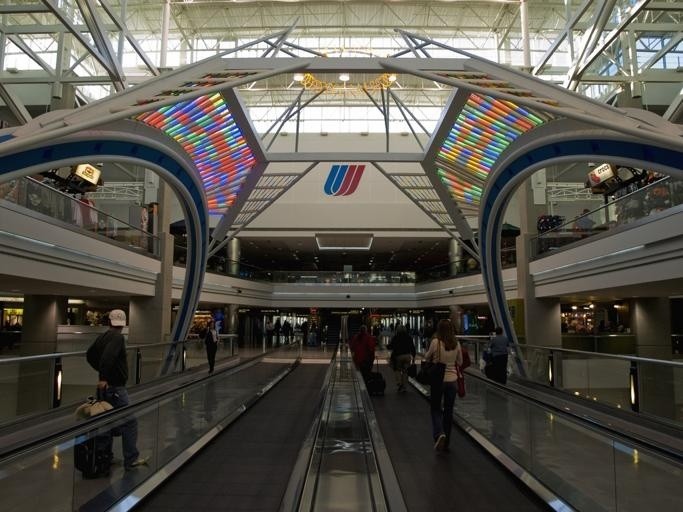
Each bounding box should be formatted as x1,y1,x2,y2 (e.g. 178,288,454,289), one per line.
131,456,150,466
434,434,446,450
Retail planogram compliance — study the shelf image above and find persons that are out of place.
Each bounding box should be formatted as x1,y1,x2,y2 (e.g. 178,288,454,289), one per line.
0,321,24,351
570,208,596,241
139,207,147,232
199,319,221,374
87,308,151,467
346,312,620,396
424,318,464,452
266,316,328,350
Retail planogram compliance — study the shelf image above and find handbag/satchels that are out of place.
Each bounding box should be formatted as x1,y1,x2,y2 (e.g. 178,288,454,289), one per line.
86,334,105,370
418,337,446,385
456,364,465,397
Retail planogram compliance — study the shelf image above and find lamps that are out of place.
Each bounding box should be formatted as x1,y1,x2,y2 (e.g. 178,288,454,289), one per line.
289,28,401,97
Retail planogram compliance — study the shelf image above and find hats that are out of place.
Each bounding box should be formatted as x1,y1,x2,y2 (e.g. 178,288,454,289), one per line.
109,309,127,326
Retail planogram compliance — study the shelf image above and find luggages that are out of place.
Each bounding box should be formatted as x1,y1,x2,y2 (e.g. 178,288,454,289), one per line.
364,357,385,391
74,388,113,469
